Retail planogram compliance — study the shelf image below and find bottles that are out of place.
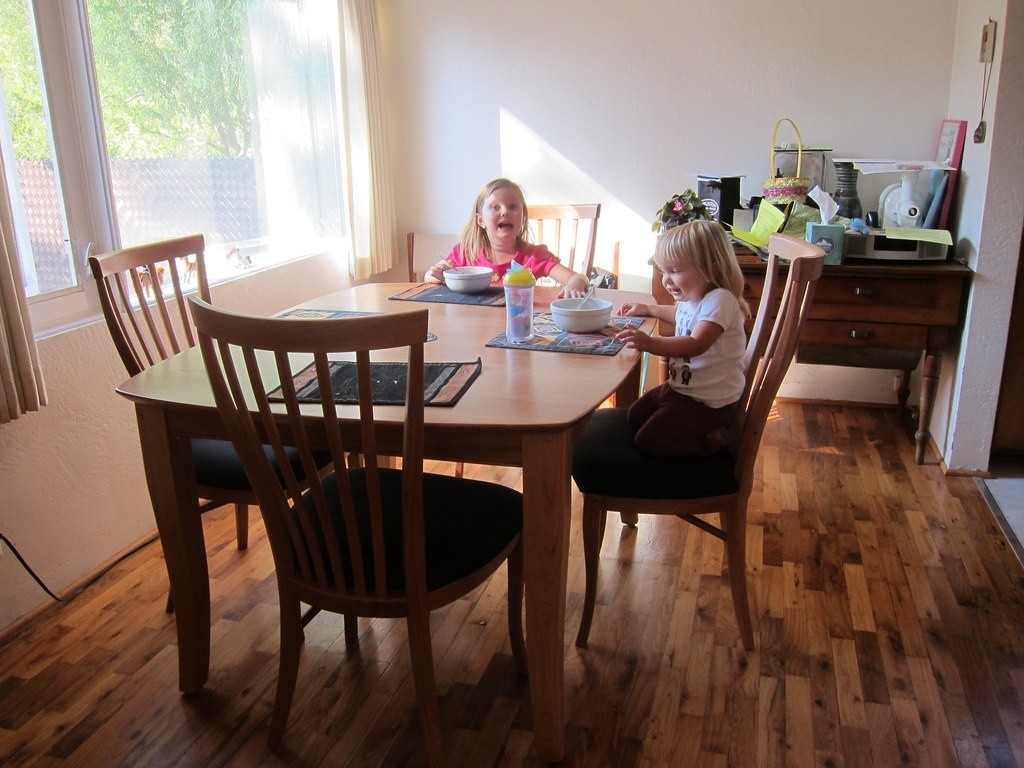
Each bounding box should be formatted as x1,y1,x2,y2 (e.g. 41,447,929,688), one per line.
503,256,536,342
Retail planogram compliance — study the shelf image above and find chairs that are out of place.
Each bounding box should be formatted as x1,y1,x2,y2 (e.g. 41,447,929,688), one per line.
88,233,353,614
406,203,599,286
572,232,827,652
187,294,529,768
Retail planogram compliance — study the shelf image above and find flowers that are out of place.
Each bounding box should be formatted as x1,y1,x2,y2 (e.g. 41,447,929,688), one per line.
651,190,712,234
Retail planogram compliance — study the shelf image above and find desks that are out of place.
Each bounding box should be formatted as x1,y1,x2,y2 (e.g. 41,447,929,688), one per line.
115,281,665,763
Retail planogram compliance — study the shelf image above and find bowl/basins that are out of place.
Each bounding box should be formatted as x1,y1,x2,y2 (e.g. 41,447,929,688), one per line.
443,266,493,293
551,298,613,333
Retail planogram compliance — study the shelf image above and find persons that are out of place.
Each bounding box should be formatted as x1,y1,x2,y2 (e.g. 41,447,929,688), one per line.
424,179,588,300
617,220,753,453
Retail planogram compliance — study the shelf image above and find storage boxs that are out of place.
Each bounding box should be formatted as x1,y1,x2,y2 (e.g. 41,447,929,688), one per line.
806,222,844,265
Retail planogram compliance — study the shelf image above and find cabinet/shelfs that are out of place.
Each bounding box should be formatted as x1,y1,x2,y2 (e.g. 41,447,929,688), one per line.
648,241,971,465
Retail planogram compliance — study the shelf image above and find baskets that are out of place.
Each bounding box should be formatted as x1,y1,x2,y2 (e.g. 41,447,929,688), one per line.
762,118,809,205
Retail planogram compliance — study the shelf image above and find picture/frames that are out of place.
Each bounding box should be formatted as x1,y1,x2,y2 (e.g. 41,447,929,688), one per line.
936,118,968,231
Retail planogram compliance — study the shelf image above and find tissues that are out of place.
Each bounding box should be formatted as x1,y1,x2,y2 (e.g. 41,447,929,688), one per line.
805,185,845,265
